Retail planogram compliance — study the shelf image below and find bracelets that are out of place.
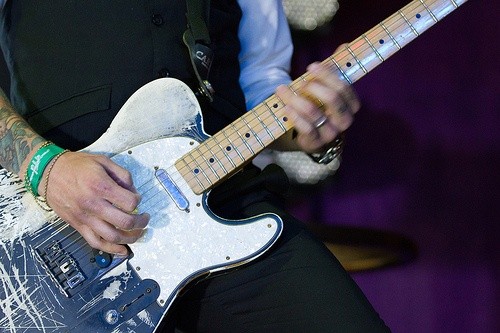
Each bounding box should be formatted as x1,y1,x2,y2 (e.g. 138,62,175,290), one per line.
25,141,69,212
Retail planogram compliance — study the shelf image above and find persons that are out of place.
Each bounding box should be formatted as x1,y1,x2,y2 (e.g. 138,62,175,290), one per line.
0,0,391,333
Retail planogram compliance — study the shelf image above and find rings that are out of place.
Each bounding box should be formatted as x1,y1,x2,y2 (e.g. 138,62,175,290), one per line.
311,116,328,129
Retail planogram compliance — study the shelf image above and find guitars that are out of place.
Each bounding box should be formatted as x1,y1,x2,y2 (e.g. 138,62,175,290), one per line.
0,0,469,333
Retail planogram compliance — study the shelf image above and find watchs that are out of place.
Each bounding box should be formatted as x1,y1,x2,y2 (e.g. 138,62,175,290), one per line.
308,137,344,164
